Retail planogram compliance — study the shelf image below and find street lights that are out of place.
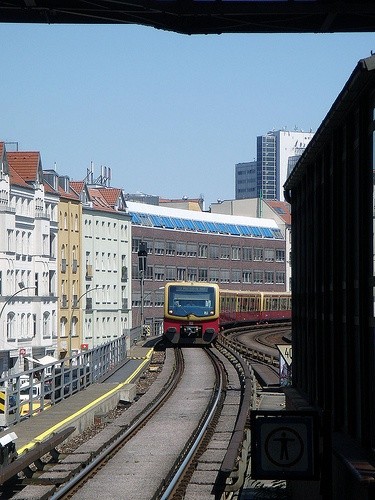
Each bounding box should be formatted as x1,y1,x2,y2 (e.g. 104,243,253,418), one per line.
136,286,165,339
68,286,110,356
0,286,38,317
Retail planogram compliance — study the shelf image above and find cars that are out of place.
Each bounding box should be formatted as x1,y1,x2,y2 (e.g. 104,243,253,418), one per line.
5,356,106,416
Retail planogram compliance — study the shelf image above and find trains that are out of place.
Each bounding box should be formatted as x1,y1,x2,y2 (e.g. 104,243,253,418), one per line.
162,281,291,348
